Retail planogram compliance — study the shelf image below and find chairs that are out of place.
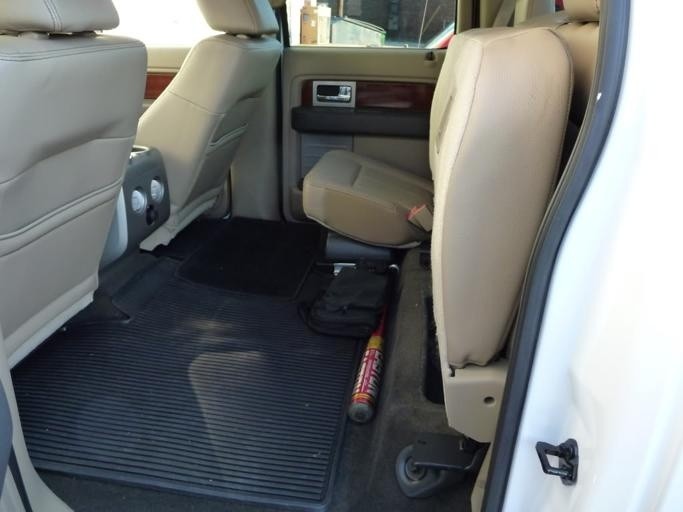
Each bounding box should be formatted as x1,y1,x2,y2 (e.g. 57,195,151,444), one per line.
1,0,149,368
301,2,604,442
128,2,282,254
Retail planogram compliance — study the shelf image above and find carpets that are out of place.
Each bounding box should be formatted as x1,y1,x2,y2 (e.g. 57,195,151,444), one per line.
2,281,360,510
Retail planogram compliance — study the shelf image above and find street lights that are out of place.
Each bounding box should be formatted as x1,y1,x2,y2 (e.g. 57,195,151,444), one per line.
295,262,391,341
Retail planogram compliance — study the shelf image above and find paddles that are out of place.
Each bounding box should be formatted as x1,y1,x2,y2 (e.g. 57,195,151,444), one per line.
2,281,360,510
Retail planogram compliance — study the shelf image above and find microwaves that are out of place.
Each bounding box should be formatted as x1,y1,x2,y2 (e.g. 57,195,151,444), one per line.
347,264,400,423
